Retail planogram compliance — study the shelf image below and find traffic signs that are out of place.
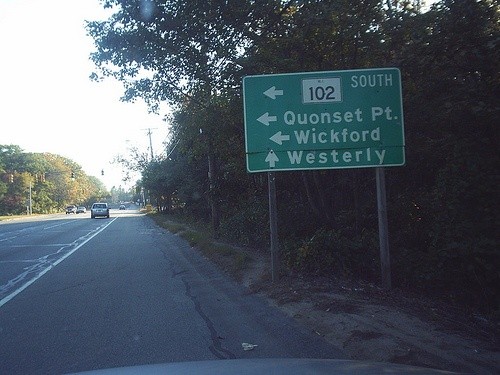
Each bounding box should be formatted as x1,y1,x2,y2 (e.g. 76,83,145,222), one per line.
243,67,406,174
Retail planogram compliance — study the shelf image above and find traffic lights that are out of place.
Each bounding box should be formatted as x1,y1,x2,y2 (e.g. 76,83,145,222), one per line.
102,170,104,176
9,174,14,183
71,172,74,178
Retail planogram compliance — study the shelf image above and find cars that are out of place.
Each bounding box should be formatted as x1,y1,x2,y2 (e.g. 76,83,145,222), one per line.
91,202,111,219
78,206,86,213
119,205,125,210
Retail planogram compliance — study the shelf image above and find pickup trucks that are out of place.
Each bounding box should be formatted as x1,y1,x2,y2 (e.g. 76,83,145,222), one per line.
65,205,77,215
